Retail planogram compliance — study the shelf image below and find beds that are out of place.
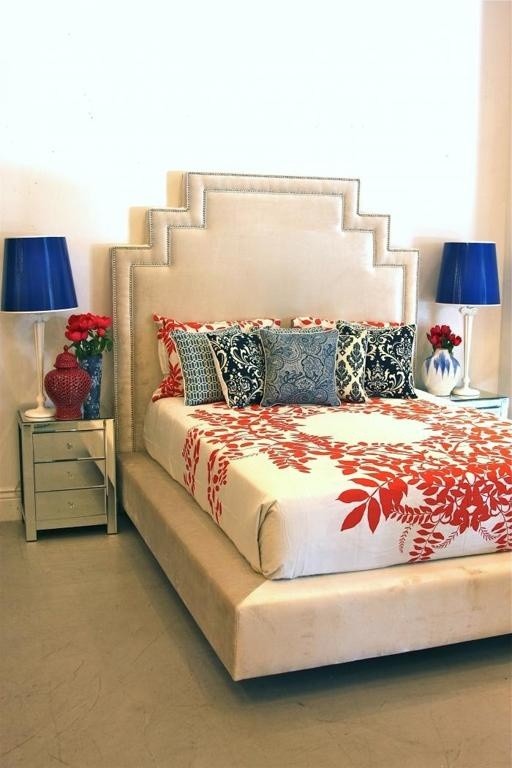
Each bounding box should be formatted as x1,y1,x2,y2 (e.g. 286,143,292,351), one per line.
108,172,511,682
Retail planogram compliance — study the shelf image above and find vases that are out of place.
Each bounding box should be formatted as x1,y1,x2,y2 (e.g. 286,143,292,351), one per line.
423,348,458,396
75,354,102,419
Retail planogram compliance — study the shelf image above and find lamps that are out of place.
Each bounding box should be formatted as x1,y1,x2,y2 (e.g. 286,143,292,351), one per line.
2,234,78,422
434,241,500,400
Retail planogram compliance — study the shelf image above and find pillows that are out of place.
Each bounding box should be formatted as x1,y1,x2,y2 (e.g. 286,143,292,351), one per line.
149,312,418,413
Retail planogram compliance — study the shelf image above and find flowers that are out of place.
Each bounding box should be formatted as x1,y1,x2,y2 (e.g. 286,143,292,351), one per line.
425,323,462,353
63,313,114,359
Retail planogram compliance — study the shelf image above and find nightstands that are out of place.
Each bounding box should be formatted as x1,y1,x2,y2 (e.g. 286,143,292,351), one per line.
435,388,508,415
20,407,118,544
